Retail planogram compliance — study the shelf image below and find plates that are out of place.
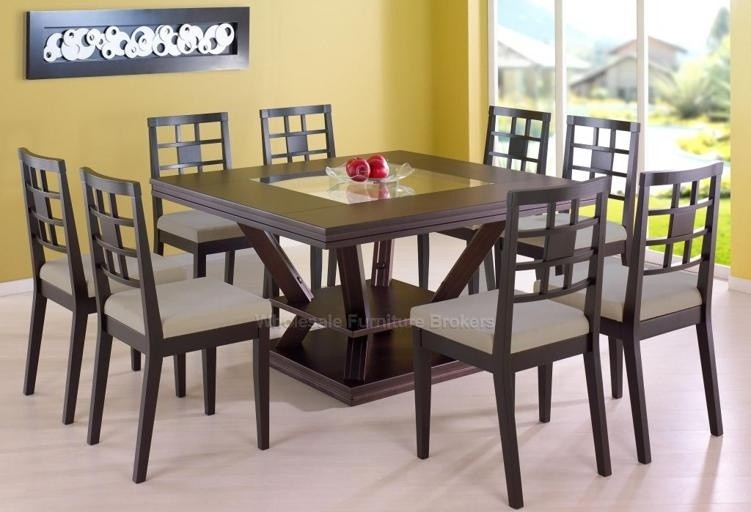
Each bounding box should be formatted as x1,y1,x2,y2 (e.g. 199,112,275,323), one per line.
326,161,414,182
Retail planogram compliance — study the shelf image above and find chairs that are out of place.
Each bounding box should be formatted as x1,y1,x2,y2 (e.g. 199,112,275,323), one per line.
494,115,641,287
80,167,272,483
417,106,551,295
409,177,612,509
260,104,336,299
533,162,724,465
147,112,279,326
17,147,187,425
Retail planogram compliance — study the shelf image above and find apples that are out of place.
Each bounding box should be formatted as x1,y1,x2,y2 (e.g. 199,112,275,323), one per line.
346,154,389,182
